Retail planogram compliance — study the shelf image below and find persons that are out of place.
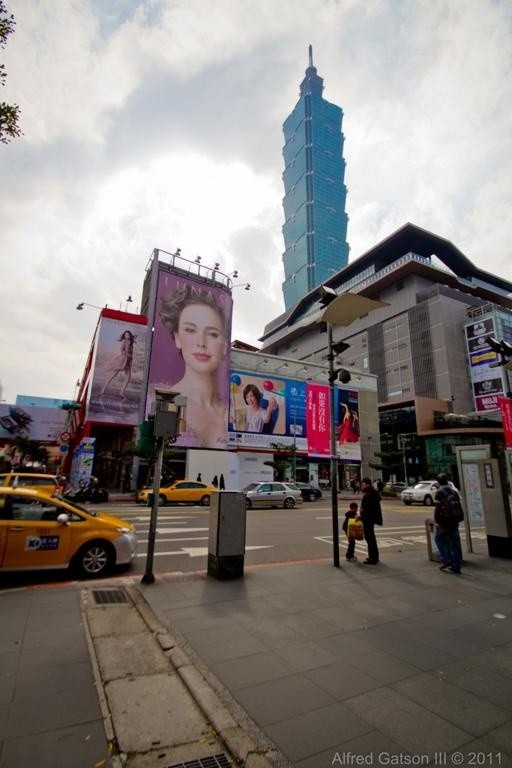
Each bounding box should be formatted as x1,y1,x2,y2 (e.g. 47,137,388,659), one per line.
148,281,231,446
355,477,383,566
228,383,277,436
283,474,317,489
212,475,218,487
433,470,464,575
100,330,135,401
219,473,226,490
196,473,202,481
339,401,358,443
349,473,383,499
342,502,358,561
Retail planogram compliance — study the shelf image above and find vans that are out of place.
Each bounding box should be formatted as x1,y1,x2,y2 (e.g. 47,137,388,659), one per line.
0,472,74,501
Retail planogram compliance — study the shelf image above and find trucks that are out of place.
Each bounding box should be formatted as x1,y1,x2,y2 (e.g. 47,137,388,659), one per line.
233,480,304,511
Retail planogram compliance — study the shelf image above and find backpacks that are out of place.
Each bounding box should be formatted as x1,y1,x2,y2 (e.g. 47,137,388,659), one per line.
443,491,464,523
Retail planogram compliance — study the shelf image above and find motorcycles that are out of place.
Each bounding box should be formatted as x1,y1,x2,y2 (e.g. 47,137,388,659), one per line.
76,480,109,505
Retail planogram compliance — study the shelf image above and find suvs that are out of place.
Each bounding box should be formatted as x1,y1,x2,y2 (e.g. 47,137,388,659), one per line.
403,480,461,508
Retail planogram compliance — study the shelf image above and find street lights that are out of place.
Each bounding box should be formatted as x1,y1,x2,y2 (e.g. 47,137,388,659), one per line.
484,336,512,398
317,284,352,567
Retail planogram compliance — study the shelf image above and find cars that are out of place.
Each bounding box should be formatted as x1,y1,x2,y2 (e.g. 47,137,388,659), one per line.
137,480,217,506
0,486,137,577
385,481,406,488
288,479,322,502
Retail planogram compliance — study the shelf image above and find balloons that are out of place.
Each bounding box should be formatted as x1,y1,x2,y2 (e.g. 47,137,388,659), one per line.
263,381,273,391
231,374,242,395
